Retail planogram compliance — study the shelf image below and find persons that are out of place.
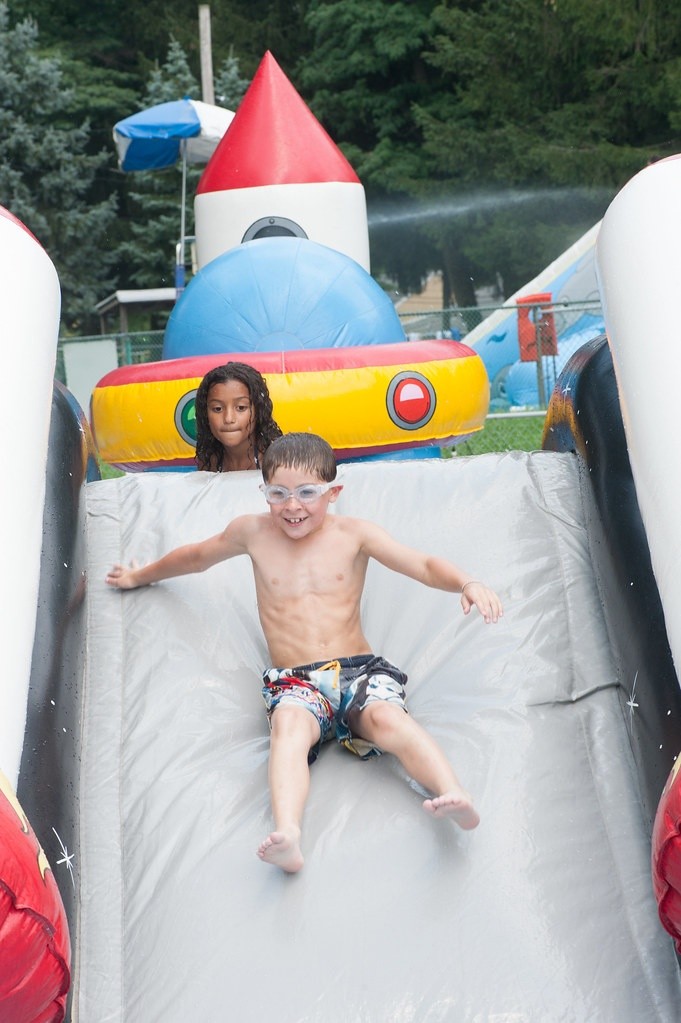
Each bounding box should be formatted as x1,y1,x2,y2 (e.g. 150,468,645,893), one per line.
193,362,283,472
108,432,505,872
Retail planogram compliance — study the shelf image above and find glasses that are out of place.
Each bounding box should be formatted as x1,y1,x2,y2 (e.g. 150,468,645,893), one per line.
259,473,344,503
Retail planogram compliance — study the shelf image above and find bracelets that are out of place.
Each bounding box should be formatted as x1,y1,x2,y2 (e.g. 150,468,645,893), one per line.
461,581,483,591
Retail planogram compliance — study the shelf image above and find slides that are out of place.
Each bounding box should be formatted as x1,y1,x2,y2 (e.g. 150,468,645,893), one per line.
0,153,681,1023
